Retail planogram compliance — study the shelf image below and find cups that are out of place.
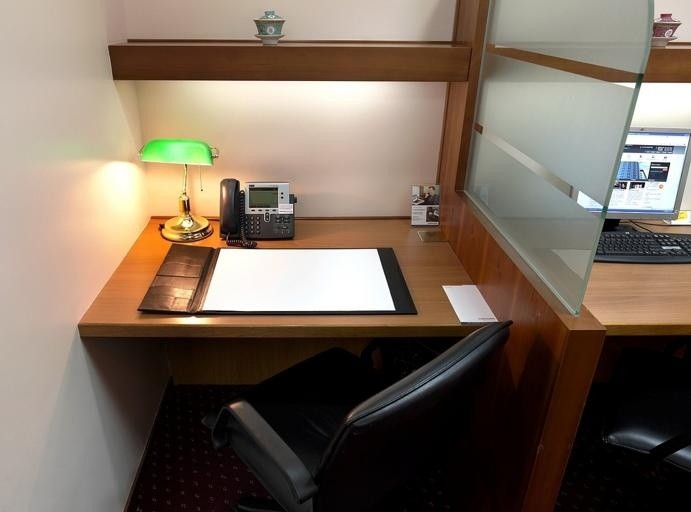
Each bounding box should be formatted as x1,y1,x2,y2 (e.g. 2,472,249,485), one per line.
253,10,287,35
653,12,681,37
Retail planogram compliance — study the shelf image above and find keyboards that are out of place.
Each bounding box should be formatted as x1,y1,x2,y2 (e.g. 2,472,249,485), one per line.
593,231,691,265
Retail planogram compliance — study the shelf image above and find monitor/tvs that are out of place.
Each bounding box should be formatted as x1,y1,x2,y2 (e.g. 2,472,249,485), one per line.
569,126,691,229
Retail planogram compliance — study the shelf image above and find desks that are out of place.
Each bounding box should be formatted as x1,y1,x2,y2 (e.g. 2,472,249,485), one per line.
77,218,501,340
584,216,689,335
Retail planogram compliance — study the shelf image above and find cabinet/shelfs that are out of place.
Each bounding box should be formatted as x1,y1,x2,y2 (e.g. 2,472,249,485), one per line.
643,40,690,83
108,36,474,84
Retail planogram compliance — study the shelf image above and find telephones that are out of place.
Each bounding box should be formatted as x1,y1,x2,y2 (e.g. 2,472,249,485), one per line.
219,178,298,250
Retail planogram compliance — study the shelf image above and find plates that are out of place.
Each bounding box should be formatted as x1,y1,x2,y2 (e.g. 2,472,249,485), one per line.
651,37,679,46
254,33,285,45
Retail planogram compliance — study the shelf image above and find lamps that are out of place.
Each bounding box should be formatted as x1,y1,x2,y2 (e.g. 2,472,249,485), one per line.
138,138,222,243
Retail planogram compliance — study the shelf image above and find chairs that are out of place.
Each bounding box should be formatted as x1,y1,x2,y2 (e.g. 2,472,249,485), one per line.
214,319,516,512
601,389,691,497
